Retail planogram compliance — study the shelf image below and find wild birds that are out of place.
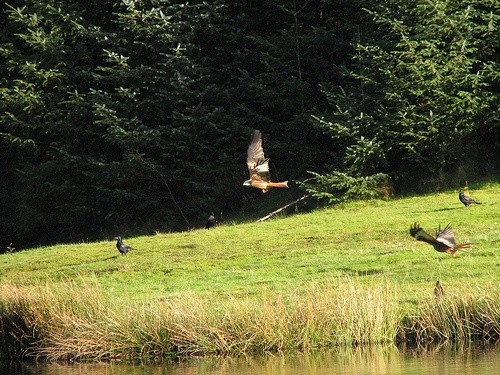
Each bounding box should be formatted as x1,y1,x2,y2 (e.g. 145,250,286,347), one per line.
114,235,138,258
242,129,290,194
205,212,216,231
409,221,475,257
459,189,482,208
